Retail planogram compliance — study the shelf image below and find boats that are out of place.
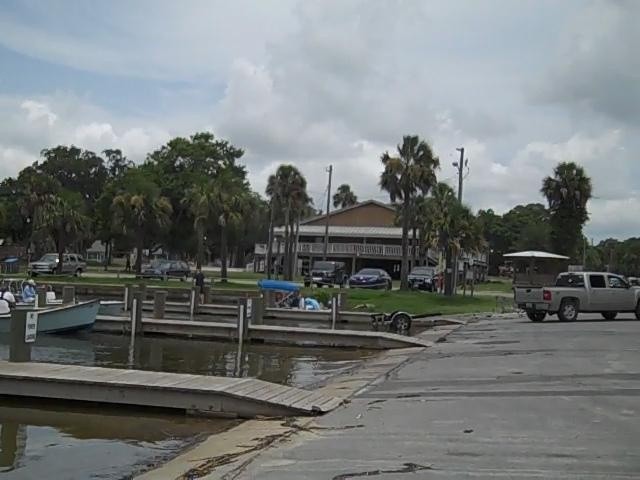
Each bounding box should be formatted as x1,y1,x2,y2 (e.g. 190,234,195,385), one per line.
258,279,389,322
0,298,101,335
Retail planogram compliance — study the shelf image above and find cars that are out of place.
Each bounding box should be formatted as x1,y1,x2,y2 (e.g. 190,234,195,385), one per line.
136,259,191,282
28,252,88,278
304,261,443,292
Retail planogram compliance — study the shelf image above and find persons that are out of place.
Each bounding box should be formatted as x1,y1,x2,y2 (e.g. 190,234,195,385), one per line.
2,285,17,304
21,279,38,305
275,290,302,308
193,267,205,304
45,285,56,303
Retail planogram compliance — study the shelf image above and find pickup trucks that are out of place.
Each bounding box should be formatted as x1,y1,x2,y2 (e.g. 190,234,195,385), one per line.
514,271,640,322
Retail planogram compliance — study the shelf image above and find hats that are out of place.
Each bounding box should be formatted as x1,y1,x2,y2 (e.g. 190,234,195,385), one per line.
28,279,36,285
